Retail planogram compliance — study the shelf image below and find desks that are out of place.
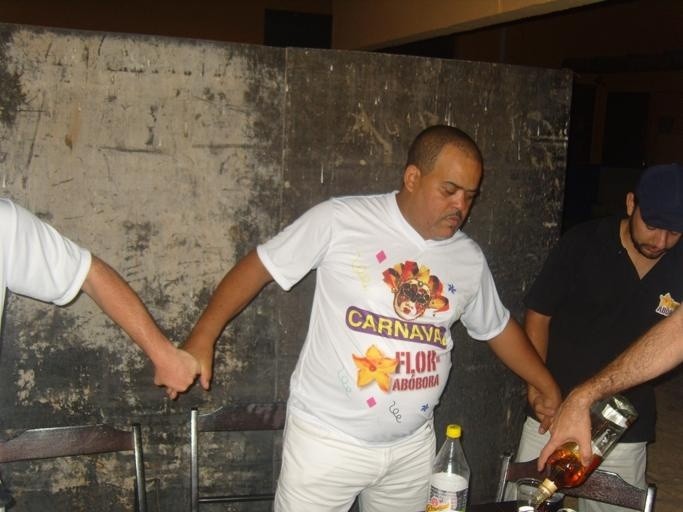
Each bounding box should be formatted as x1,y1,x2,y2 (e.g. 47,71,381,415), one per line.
418,500,577,512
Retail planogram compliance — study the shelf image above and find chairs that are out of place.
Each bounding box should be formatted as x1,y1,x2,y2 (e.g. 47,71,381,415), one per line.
190,401,286,512
0,423,148,512
495,454,657,512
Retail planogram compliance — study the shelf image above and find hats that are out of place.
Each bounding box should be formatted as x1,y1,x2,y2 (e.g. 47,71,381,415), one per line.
633,164,682,235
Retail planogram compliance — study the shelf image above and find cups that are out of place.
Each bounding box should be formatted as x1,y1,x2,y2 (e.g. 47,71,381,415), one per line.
514,478,540,510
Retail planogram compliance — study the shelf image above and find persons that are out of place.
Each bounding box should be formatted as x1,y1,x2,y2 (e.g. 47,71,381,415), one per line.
0,196,199,393
503,163,683,510
535,303,683,473
165,124,563,511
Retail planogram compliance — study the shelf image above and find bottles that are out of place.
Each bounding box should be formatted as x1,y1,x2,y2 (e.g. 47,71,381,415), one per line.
427,422,470,511
527,391,639,510
